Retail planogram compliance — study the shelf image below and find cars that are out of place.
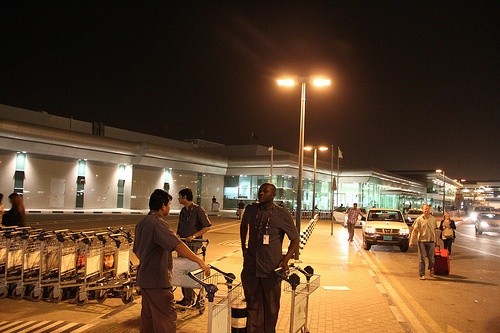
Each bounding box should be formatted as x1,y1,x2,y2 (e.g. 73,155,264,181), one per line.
404,209,423,224
333,206,367,227
475,212,500,234
470,205,496,223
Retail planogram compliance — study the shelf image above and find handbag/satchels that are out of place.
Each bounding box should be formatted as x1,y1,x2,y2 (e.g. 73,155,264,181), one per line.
444,228,453,238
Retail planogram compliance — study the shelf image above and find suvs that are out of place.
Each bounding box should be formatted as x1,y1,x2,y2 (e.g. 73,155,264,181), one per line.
361,208,410,253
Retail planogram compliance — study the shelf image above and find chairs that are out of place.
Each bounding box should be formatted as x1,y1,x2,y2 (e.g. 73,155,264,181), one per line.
389,215,395,219
372,214,378,218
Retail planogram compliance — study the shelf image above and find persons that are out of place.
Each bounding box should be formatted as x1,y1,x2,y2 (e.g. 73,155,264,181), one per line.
409,203,440,280
240,182,300,333
439,212,456,255
175,188,212,309
437,203,468,212
133,189,211,333
344,203,366,241
0,193,4,227
340,204,344,207
402,202,411,213
212,196,216,212
2,193,26,227
315,205,318,209
238,200,257,220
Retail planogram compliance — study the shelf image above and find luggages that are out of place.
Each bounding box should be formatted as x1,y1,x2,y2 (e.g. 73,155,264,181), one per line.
434,246,450,275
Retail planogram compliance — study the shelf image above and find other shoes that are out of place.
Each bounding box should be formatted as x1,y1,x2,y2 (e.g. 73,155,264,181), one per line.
176,298,191,306
196,296,205,309
430,269,434,276
420,276,424,279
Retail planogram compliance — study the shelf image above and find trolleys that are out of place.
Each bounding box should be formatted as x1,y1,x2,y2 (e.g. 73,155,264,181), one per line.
186,261,320,333
0,224,210,315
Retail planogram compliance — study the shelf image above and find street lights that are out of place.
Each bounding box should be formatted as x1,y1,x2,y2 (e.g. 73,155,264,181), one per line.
435,168,447,215
303,145,329,219
276,73,333,264
453,178,466,217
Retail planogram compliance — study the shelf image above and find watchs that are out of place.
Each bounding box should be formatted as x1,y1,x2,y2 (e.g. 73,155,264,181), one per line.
192,235,196,238
